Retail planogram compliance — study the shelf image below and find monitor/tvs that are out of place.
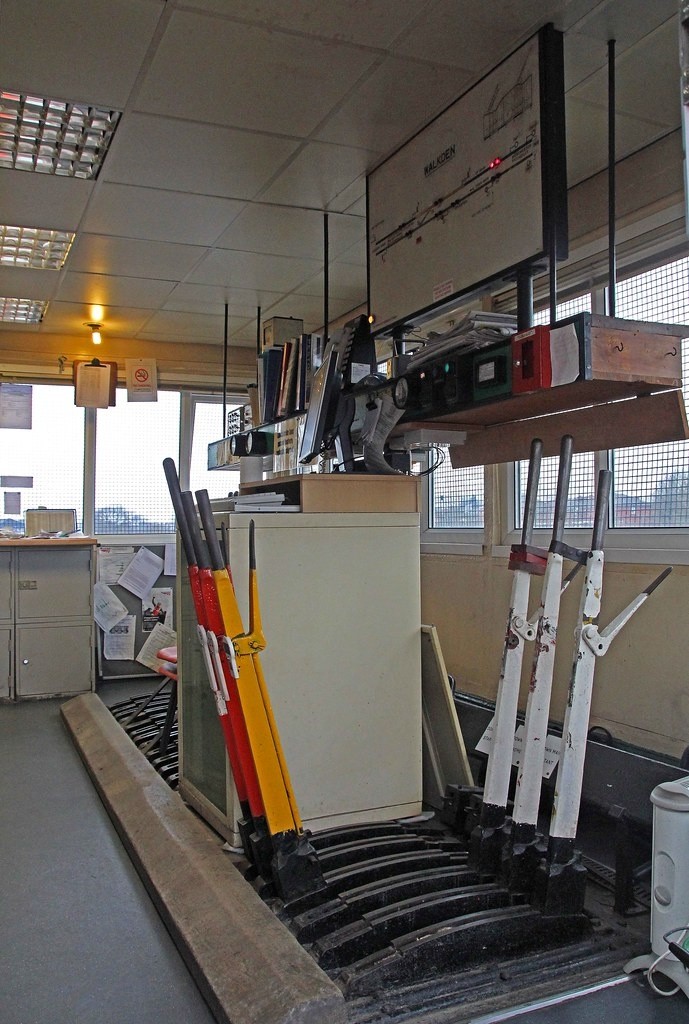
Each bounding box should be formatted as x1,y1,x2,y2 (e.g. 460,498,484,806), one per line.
295,351,338,464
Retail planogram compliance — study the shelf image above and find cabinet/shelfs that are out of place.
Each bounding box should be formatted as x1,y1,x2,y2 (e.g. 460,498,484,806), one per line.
1,539,98,708
171,512,428,846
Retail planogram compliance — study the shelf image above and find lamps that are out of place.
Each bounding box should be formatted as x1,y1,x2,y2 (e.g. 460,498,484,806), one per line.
83,322,104,344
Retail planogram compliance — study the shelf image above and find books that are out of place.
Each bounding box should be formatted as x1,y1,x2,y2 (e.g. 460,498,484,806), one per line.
209,491,285,506
257,332,326,423
